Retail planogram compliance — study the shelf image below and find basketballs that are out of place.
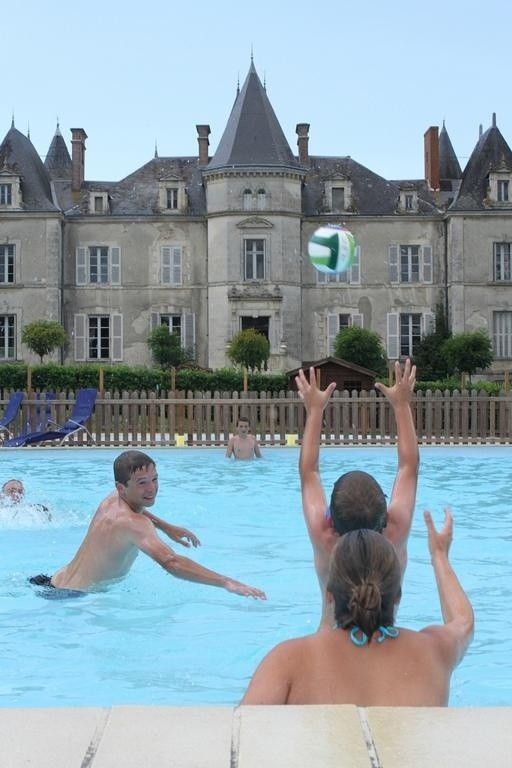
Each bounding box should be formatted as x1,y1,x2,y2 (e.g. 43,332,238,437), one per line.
307,224,356,275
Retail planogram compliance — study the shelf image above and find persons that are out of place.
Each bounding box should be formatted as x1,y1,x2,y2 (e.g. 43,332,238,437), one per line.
241,509,474,708
3,480,23,506
224,418,262,459
31,450,268,601
295,359,420,630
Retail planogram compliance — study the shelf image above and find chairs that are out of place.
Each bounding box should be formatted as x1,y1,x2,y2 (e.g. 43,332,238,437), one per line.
0,388,101,448
